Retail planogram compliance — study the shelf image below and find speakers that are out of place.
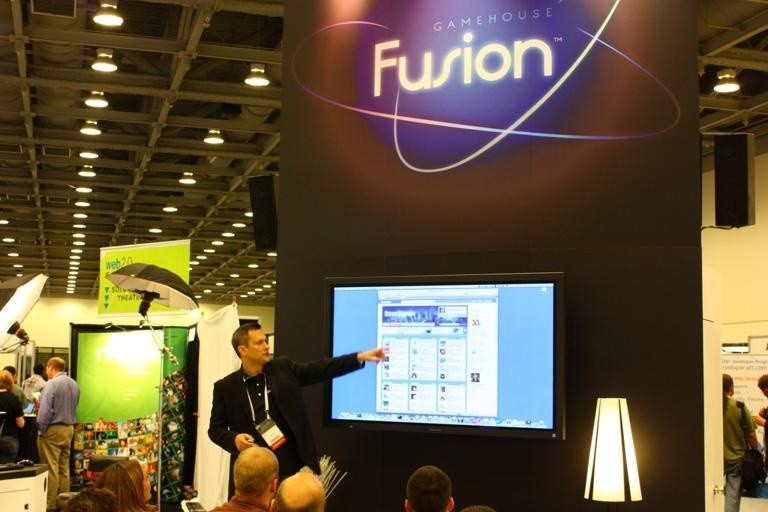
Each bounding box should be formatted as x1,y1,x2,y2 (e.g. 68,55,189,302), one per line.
714,132,756,227
246,173,279,254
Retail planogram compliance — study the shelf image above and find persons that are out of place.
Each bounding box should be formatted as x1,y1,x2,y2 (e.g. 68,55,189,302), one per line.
206,320,386,502
35,357,80,511
751,375,767,476
404,465,454,511
62,486,119,511
724,373,758,512
93,458,160,511
0,361,70,472
269,472,327,511
209,445,280,512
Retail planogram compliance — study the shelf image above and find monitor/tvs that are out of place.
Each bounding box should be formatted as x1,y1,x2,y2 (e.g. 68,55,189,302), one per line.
321,272,566,440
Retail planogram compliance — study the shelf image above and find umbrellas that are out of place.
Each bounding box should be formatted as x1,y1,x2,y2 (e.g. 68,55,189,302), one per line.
0,271,50,348
107,263,199,313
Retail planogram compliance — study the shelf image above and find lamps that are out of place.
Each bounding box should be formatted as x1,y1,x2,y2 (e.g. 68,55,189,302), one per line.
244,62,272,87
582,396,644,512
163,203,178,213
189,210,277,299
77,164,96,177
65,185,94,296
78,148,99,159
79,119,102,135
85,91,109,109
148,225,163,234
713,69,741,94
90,47,118,72
0,217,23,278
203,128,224,144
178,172,197,185
92,0,124,27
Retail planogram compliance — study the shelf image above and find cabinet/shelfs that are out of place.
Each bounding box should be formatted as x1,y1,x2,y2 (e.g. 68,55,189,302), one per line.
0,463,50,512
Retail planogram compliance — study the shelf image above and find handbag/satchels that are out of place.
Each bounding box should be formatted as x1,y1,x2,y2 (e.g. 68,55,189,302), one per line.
744,448,766,490
